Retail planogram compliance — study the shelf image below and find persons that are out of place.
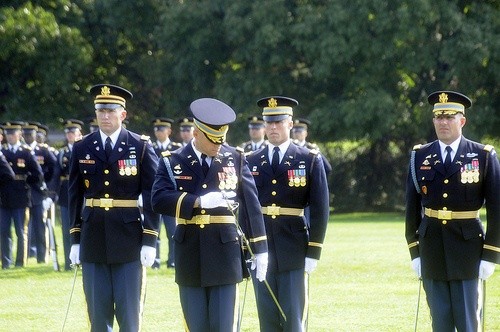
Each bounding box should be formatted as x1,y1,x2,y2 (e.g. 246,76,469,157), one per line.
238,116,269,152
177,117,194,147
152,98,268,332
292,119,332,225
151,117,176,269
243,96,329,332
405,91,500,332
0,117,99,271
67,85,161,332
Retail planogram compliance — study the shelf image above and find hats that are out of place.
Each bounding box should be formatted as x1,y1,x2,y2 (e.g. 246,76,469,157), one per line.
256,95,298,122
0,121,49,136
247,117,265,129
88,119,99,128
62,119,84,133
90,84,133,110
177,118,194,131
152,117,174,132
290,118,312,132
190,97,237,144
122,120,129,129
427,91,471,116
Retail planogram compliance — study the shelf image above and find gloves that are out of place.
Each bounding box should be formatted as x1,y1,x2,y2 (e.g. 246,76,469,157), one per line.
42,197,52,210
412,257,422,281
250,253,269,283
479,260,496,281
140,245,156,268
199,190,230,209
305,256,318,275
69,244,80,266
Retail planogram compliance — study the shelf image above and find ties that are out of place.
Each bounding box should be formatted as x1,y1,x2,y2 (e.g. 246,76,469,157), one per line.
201,153,210,179
444,146,453,171
104,136,112,159
271,147,280,174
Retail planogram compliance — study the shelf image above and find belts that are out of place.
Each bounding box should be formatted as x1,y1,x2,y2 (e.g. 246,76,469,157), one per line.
176,215,236,224
86,198,138,208
424,208,480,220
261,206,305,217
